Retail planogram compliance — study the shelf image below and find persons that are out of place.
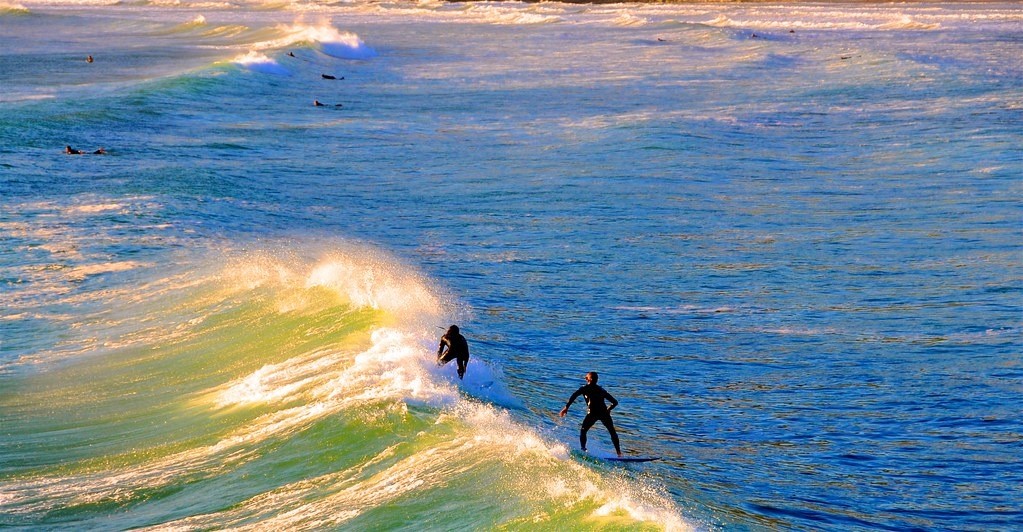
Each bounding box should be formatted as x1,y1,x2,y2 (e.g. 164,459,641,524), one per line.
321,73,345,79
65,146,105,154
87,55,93,63
287,52,295,57
560,371,623,457
314,101,343,106
435,323,470,379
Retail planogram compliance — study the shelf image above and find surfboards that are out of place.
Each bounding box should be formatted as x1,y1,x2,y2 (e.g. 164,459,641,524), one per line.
606,455,662,463
479,380,495,388
